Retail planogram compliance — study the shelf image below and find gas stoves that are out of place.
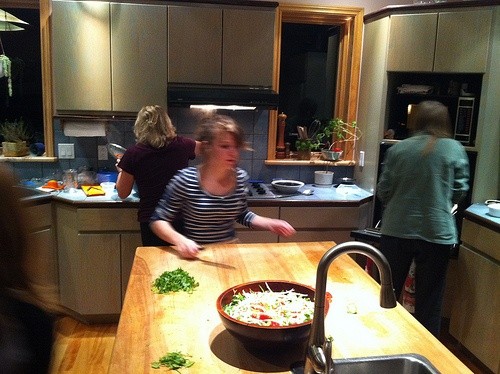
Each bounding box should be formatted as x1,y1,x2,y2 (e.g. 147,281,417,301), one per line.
243,181,275,199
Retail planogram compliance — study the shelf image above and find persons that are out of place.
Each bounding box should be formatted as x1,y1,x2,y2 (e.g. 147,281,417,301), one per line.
0,161,55,374
376,101,470,340
116,105,254,247
149,115,296,259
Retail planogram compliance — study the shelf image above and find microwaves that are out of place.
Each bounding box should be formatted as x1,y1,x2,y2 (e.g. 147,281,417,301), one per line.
394,91,476,143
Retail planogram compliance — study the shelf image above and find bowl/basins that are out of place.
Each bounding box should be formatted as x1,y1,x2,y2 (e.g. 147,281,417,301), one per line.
487,205,500,217
218,281,329,354
271,180,304,193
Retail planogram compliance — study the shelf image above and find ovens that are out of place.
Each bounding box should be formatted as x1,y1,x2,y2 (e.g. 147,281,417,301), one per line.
373,141,478,261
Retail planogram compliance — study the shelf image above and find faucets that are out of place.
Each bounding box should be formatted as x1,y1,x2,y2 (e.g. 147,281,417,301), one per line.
302,241,399,374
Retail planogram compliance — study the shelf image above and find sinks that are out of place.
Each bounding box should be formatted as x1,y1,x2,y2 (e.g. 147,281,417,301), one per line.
292,355,439,374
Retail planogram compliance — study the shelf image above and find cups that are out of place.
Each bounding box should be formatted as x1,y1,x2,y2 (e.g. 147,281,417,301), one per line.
100,182,115,196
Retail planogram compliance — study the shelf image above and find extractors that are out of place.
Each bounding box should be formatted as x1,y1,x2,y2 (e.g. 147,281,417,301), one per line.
166,82,279,112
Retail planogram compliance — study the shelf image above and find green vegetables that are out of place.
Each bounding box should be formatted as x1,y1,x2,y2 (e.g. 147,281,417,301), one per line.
223,289,315,325
151,268,200,295
151,352,196,370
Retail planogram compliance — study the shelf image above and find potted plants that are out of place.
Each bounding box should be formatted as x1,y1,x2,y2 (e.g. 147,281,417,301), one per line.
295,118,362,160
0,120,27,156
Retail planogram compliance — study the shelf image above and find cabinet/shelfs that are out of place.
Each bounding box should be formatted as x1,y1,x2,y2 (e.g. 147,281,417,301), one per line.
20,200,142,325
363,2,500,210
51,0,279,115
449,218,500,374
232,202,371,262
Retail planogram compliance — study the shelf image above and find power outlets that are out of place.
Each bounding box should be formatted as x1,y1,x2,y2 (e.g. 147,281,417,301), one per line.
98,146,108,161
